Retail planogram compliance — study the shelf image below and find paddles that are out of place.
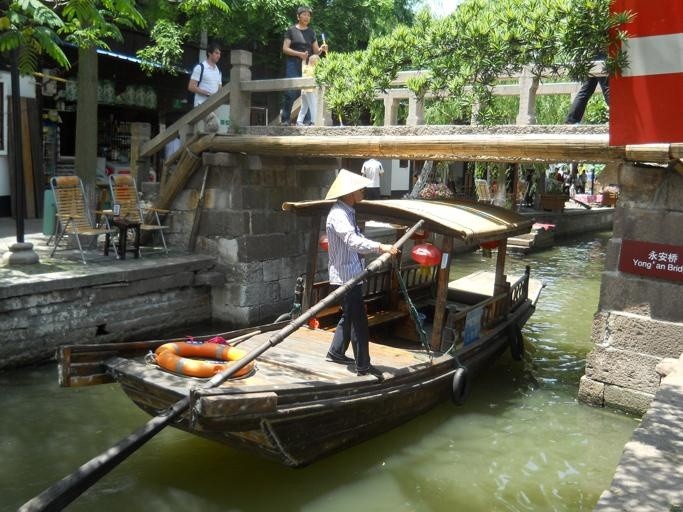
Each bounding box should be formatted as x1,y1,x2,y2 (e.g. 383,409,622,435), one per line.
16,218,423,511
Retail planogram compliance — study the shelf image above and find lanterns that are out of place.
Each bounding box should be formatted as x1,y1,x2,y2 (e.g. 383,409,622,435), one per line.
319,237,328,251
479,240,497,259
410,239,442,276
412,230,428,244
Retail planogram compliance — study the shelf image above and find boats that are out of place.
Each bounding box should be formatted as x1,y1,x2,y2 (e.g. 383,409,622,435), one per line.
102,197,546,470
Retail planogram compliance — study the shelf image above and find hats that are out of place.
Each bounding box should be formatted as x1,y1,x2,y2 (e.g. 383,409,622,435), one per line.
322,168,371,200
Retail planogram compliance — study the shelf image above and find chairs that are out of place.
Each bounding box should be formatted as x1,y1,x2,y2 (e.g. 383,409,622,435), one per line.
49,173,169,266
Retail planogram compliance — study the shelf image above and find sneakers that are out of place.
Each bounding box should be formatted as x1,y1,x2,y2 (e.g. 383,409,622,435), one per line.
280,121,303,126
356,366,380,376
324,351,355,363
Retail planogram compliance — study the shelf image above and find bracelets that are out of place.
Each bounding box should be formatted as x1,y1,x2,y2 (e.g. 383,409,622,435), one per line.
206,91,210,98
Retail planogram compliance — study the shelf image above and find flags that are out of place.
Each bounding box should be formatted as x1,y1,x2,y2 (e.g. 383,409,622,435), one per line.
608,1,683,145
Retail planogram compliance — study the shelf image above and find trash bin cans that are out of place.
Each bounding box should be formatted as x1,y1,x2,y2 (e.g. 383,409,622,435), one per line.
42,191,55,234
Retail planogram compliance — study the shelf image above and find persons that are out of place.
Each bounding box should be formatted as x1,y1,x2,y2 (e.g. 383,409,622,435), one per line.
278,4,330,127
293,41,327,129
324,168,404,379
521,166,596,208
561,45,609,126
187,43,225,134
360,160,385,200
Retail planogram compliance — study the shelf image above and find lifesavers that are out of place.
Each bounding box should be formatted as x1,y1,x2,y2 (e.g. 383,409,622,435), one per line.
155,341,254,376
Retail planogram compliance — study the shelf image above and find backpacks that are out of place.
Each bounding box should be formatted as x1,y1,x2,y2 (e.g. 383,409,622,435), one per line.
187,63,221,106
